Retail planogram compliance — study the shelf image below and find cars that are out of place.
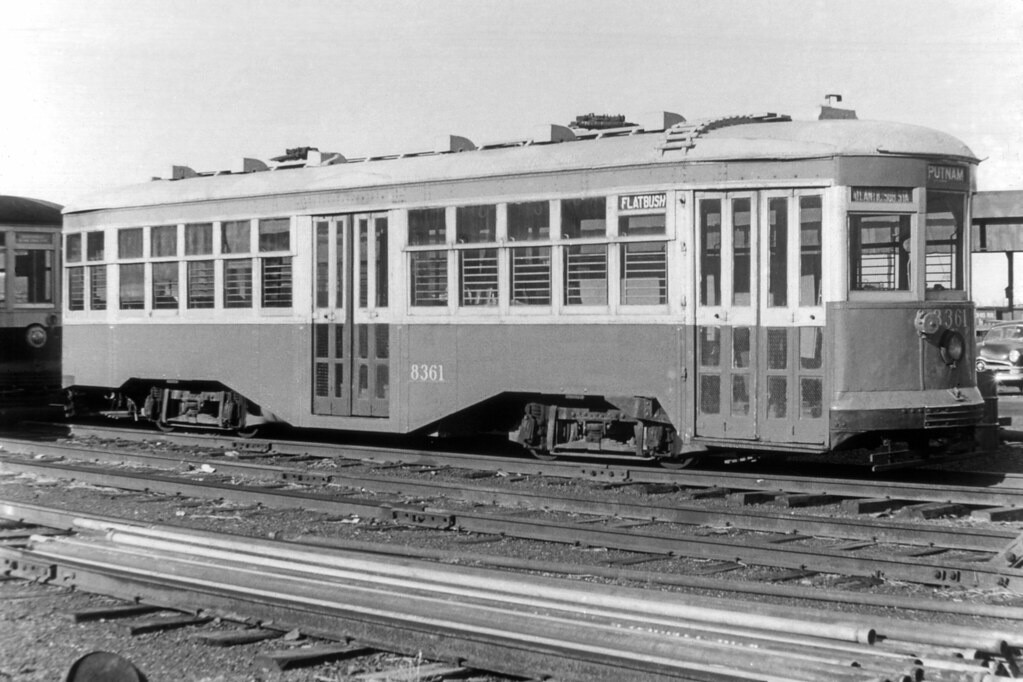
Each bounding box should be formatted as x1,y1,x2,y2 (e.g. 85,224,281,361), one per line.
976,319,1023,397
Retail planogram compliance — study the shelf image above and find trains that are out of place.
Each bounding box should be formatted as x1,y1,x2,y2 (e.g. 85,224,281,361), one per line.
0,94,985,466
